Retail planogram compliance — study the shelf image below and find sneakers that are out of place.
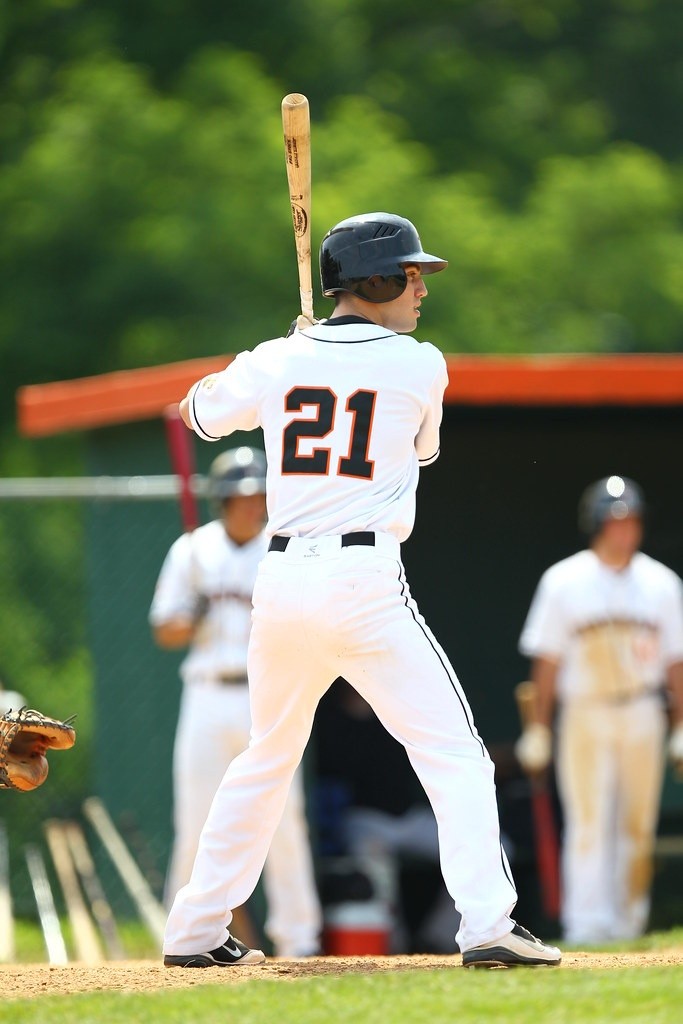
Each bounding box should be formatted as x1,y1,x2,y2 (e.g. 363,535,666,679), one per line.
165,934,266,968
461,919,562,971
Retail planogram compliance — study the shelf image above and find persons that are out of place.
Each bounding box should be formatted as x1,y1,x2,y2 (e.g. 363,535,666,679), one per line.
145,446,323,957
164,212,562,967
517,478,683,939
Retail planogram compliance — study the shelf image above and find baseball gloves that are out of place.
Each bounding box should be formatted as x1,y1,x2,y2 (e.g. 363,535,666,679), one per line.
0,705,77,792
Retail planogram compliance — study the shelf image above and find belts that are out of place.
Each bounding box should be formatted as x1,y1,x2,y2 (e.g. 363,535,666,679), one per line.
269,531,375,551
214,675,249,686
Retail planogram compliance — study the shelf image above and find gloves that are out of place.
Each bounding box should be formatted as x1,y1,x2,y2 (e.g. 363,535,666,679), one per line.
668,723,683,760
516,725,554,768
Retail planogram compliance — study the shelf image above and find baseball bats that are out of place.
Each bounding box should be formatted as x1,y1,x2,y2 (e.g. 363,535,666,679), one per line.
163,399,204,587
280,89,325,315
513,680,561,919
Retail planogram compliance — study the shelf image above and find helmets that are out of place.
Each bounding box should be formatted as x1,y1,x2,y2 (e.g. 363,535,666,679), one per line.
320,212,448,303
205,447,267,500
579,475,642,540
